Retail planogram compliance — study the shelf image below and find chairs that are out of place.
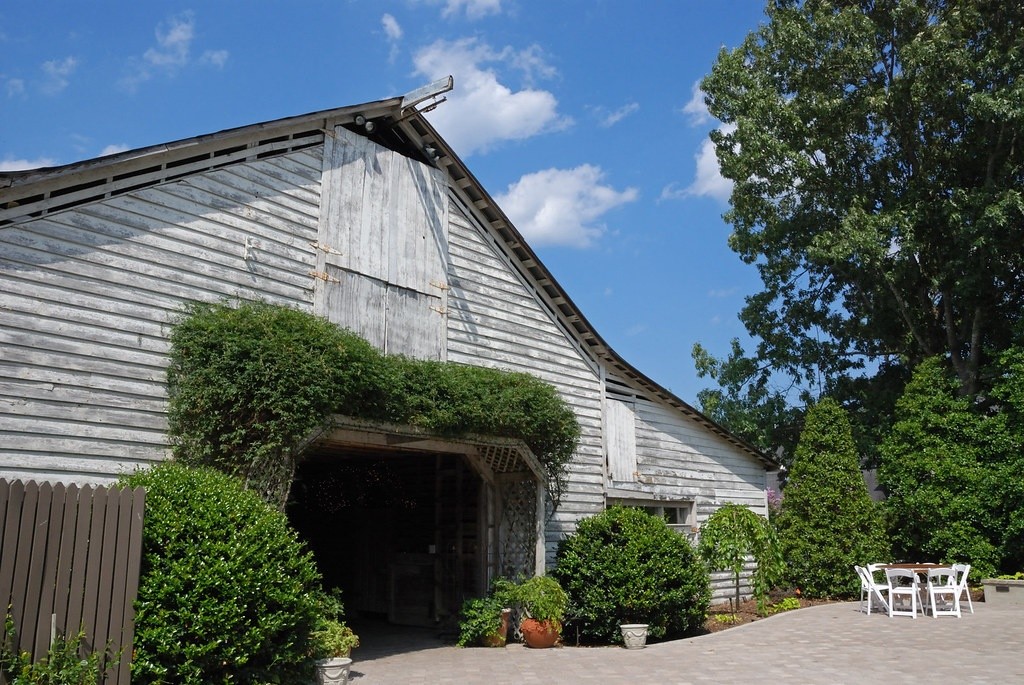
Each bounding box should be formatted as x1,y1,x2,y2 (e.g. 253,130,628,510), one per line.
855,563,974,619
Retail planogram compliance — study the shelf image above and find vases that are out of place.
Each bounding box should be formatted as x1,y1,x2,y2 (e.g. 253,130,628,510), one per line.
621,623,648,650
315,656,352,685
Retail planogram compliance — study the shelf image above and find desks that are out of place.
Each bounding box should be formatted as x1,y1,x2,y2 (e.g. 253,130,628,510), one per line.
874,564,952,608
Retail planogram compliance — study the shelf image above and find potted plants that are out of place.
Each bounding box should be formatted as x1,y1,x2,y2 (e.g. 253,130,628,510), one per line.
457,599,511,646
313,619,361,658
494,571,573,648
